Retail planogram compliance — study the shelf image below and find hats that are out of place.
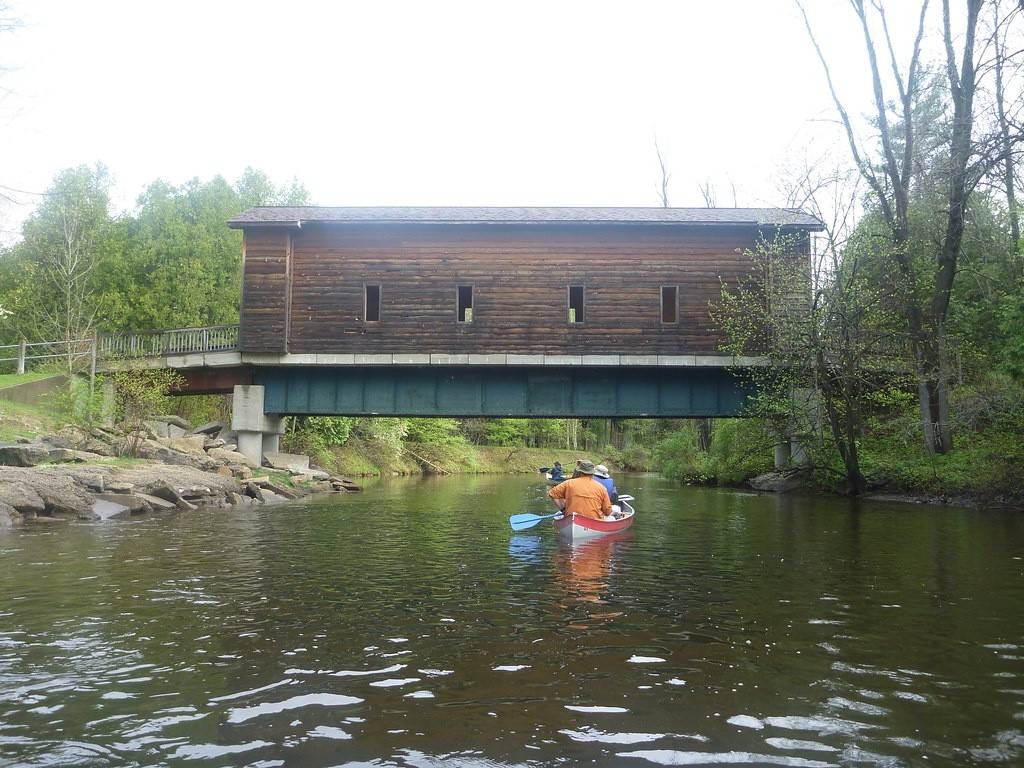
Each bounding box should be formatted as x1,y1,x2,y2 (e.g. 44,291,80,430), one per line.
554,462,560,465
576,460,595,474
594,465,610,479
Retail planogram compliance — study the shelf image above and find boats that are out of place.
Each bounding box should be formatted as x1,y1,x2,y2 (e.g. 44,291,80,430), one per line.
553,497,635,539
545,472,567,485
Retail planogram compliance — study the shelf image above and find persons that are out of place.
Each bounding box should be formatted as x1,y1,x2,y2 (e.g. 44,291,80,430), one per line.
551,461,564,480
548,461,616,521
592,464,618,505
572,459,582,479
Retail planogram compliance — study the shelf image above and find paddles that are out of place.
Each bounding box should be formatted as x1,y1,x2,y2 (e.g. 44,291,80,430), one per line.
507,492,637,534
539,466,559,474
